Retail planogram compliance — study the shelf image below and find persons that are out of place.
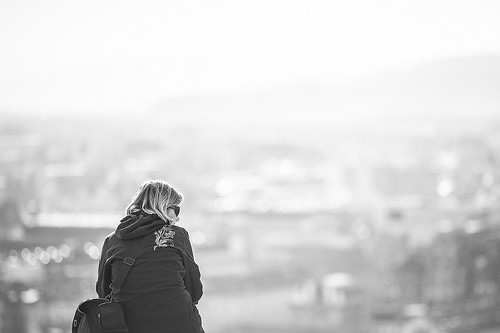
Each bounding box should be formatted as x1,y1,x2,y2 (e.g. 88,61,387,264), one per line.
96,179,205,333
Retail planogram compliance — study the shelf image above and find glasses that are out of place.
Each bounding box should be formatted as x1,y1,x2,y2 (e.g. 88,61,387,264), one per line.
167,205,180,216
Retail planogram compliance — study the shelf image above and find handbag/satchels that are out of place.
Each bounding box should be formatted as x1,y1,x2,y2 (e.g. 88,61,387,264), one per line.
72,297,129,333
